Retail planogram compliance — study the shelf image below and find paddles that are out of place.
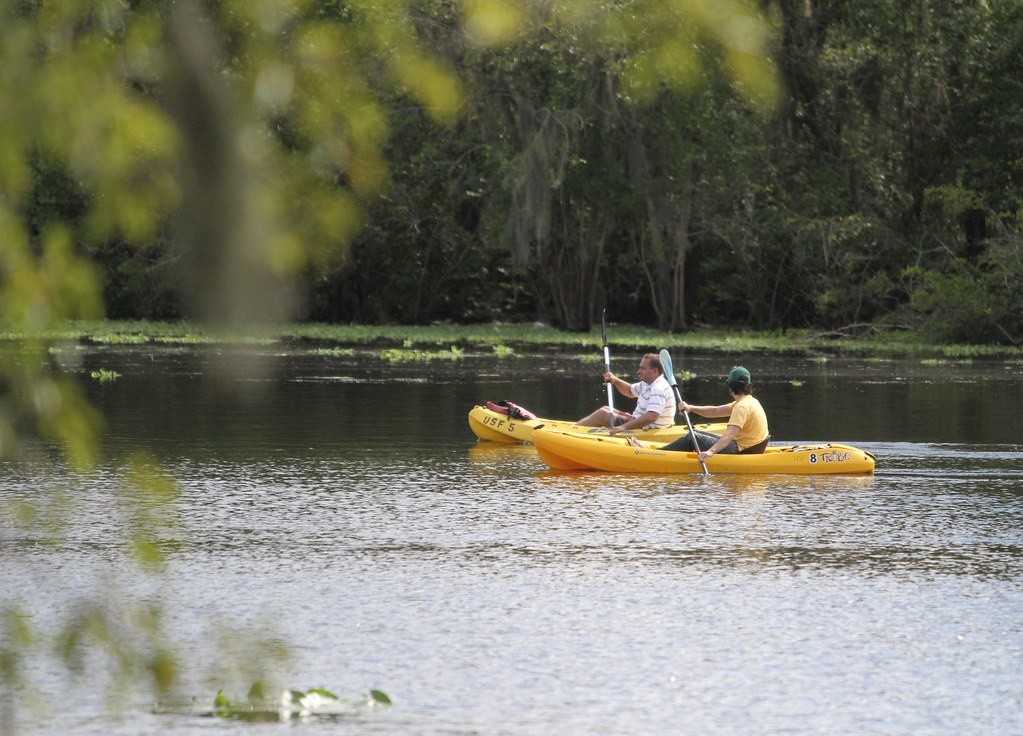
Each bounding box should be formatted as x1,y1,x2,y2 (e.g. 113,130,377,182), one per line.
658,348,709,475
600,307,614,429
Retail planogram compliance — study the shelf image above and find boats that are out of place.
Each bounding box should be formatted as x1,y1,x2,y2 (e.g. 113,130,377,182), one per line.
466,401,735,444
531,432,875,482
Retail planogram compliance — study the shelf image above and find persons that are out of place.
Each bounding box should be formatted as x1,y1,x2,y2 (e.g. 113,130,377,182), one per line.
575,354,676,437
626,366,768,463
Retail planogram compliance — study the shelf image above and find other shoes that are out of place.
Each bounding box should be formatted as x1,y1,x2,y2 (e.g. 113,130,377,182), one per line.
631,436,639,445
626,437,636,447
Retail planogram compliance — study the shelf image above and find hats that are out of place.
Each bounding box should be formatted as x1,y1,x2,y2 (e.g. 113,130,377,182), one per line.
726,366,751,385
